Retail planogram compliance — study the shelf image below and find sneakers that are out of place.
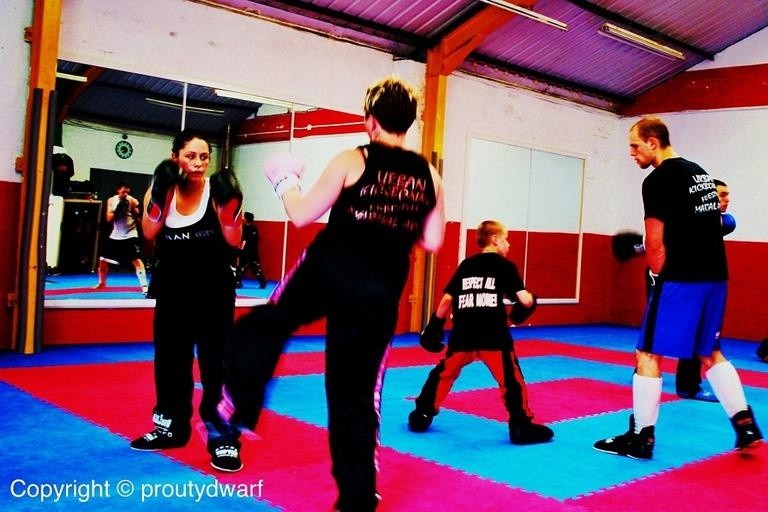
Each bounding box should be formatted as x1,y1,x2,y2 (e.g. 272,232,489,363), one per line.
408,409,433,433
129,407,192,452
730,405,764,452
207,430,243,472
677,382,719,403
508,416,554,445
592,413,656,461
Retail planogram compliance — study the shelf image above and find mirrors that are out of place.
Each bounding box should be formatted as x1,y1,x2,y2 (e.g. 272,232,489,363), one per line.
459,131,588,306
41,58,371,307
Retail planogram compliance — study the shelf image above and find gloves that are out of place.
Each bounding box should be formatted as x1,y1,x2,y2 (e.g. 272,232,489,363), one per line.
721,212,737,236
509,296,538,324
209,168,244,250
145,159,191,223
420,312,447,353
262,149,307,200
611,231,645,262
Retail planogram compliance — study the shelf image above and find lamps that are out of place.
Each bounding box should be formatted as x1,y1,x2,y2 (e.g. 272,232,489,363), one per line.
478,0,568,32
597,21,685,63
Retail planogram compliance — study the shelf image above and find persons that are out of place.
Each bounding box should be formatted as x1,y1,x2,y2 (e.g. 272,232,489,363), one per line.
234,212,267,289
89,184,149,296
122,132,246,472
678,179,735,403
404,219,553,446
219,74,448,510
591,117,767,461
